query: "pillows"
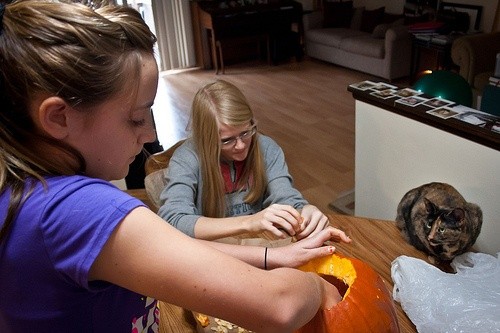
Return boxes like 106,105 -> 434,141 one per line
360,6 -> 385,32
373,18 -> 405,40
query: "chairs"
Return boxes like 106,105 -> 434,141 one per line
145,137 -> 191,221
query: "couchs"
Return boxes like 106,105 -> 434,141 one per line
451,31 -> 500,90
304,10 -> 412,84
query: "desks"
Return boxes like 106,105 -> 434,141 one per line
159,213 -> 453,333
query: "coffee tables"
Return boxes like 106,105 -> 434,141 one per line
417,41 -> 450,78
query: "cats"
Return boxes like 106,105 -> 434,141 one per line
395,182 -> 483,268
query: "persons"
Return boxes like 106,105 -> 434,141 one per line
157,79 -> 329,240
0,0 -> 352,333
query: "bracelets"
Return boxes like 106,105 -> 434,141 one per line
264,247 -> 267,270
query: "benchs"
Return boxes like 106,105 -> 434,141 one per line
210,36 -> 270,74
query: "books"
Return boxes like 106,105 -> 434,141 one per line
408,22 -> 441,36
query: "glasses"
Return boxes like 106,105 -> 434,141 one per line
215,127 -> 254,150
